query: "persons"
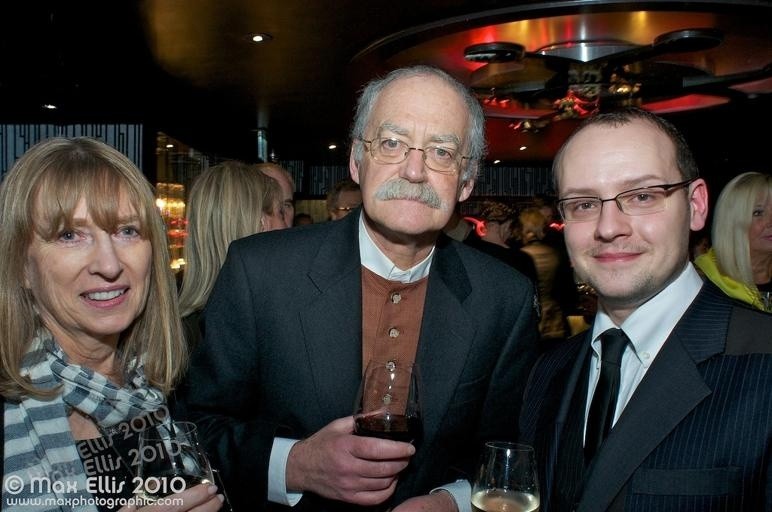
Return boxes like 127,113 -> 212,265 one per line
0,136 -> 225,512
514,105 -> 772,512
172,63 -> 542,512
444,194 -> 572,338
292,213 -> 313,226
326,179 -> 363,222
711,171 -> 772,292
252,163 -> 295,229
178,161 -> 289,318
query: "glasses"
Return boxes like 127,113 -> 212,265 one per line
557,179 -> 694,222
359,135 -> 472,173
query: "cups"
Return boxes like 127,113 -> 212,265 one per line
136,418 -> 219,503
467,438 -> 543,512
353,354 -> 425,451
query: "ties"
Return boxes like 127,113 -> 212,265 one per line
584,329 -> 630,468
